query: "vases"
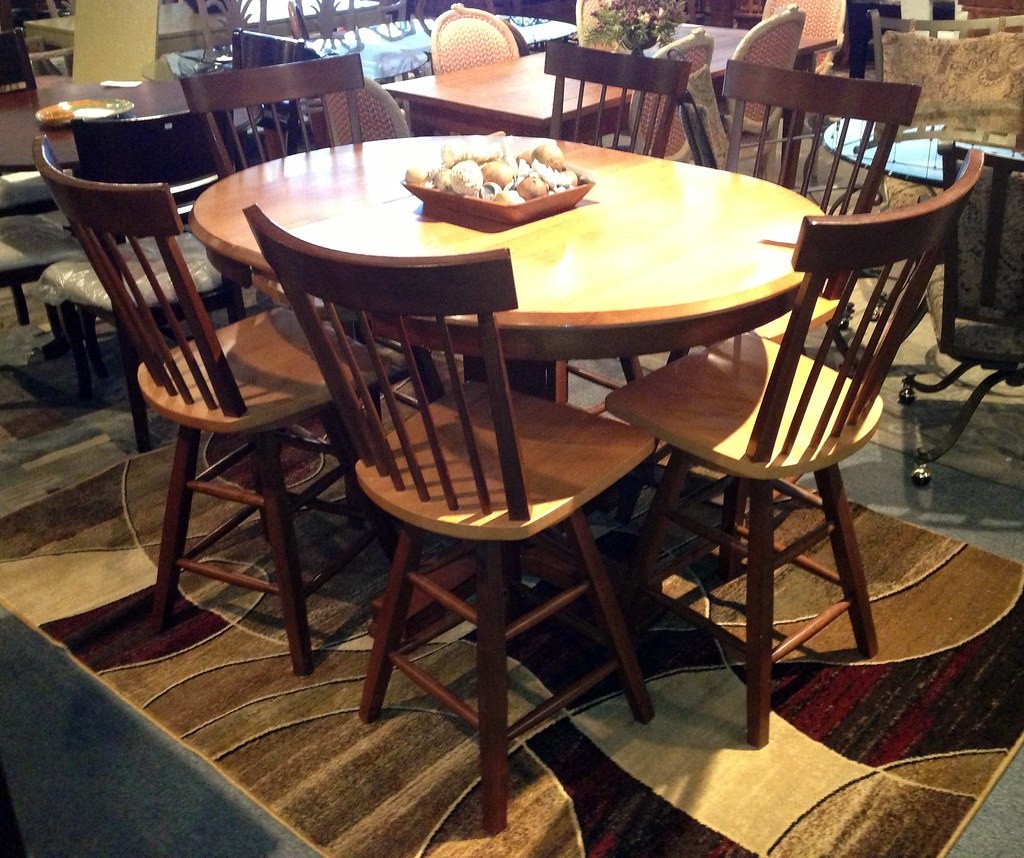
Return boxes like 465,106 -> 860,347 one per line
624,28 -> 660,57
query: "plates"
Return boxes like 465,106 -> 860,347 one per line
34,99 -> 135,127
400,176 -> 595,225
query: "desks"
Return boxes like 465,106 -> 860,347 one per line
174,13 -> 577,149
378,16 -> 839,192
0,81 -> 264,246
23,0 -> 396,96
816,99 -> 1024,363
189,134 -> 827,628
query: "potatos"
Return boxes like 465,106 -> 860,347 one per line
406,144 -> 578,208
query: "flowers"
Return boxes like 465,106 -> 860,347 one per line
579,0 -> 691,56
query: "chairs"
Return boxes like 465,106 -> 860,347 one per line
0,0 -> 1024,847
